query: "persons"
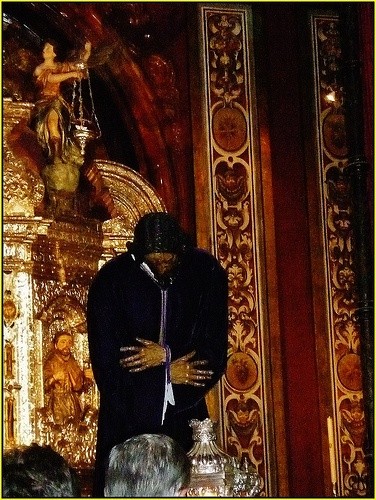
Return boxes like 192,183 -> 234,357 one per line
87,213 -> 229,499
44,331 -> 94,426
103,434 -> 191,498
0,442 -> 80,497
33,40 -> 91,163
